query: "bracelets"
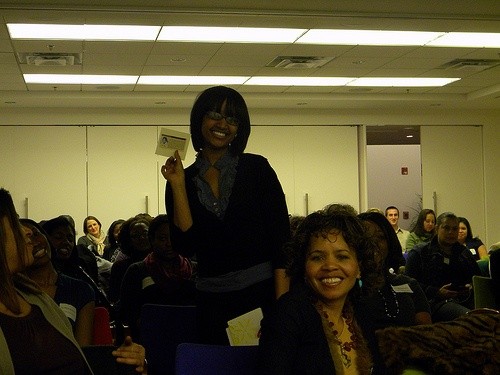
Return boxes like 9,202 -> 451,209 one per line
145,358 -> 149,368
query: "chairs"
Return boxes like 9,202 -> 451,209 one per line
472,259 -> 500,309
92,304 -> 260,375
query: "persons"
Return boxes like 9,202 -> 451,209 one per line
155,86 -> 291,347
257,212 -> 383,374
19,206 -> 488,350
0,188 -> 149,375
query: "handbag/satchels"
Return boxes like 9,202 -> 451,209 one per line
80,345 -> 141,375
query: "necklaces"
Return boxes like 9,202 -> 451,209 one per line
377,287 -> 400,318
322,301 -> 360,351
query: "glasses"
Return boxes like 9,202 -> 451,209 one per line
205,111 -> 240,126
